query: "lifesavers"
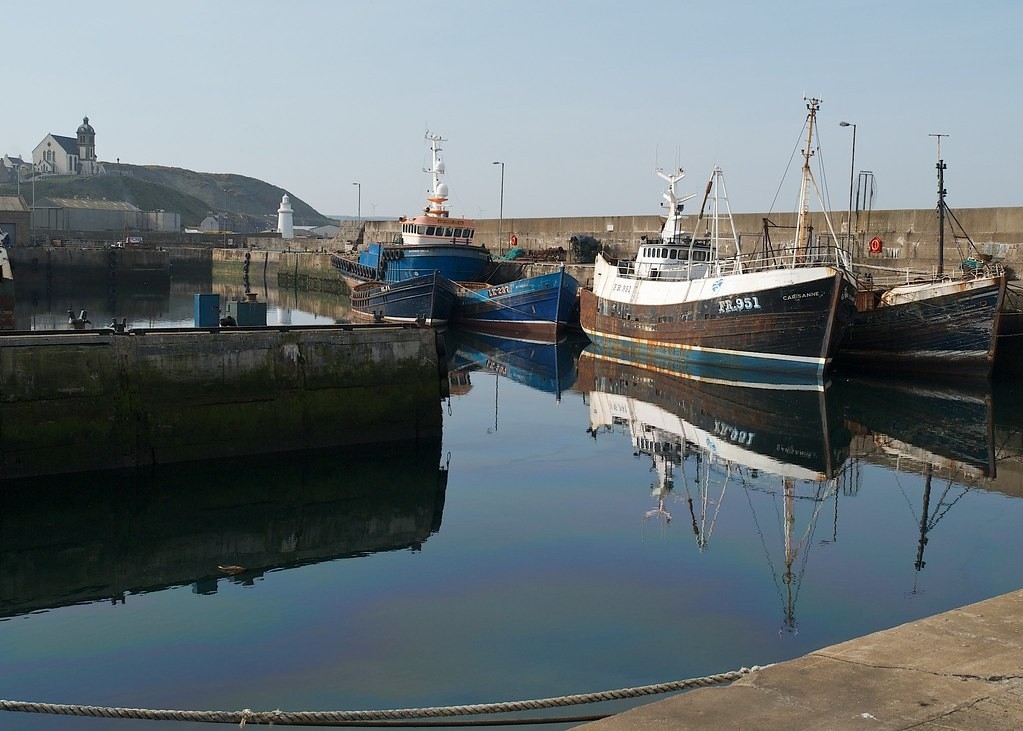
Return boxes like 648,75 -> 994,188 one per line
386,250 -> 389,260
380,260 -> 385,270
107,285 -> 119,304
32,258 -> 38,264
243,273 -> 251,294
378,270 -> 383,279
381,250 -> 386,260
108,250 -> 119,281
330,255 -> 376,280
395,250 -> 401,260
871,239 -> 879,251
512,237 -> 515,244
390,249 -> 394,260
243,252 -> 250,272
837,299 -> 858,324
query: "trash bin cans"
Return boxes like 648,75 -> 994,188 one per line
571,236 -> 595,264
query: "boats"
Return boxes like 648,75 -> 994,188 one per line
573,345 -> 854,640
577,89 -> 859,393
328,128 -> 493,293
445,329 -> 586,406
835,370 -> 998,602
350,269 -> 462,329
842,134 -> 1007,378
449,265 -> 580,344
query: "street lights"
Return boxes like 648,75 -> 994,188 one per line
353,183 -> 361,221
494,162 -> 504,257
224,190 -> 227,248
32,163 -> 37,247
840,121 -> 855,264
